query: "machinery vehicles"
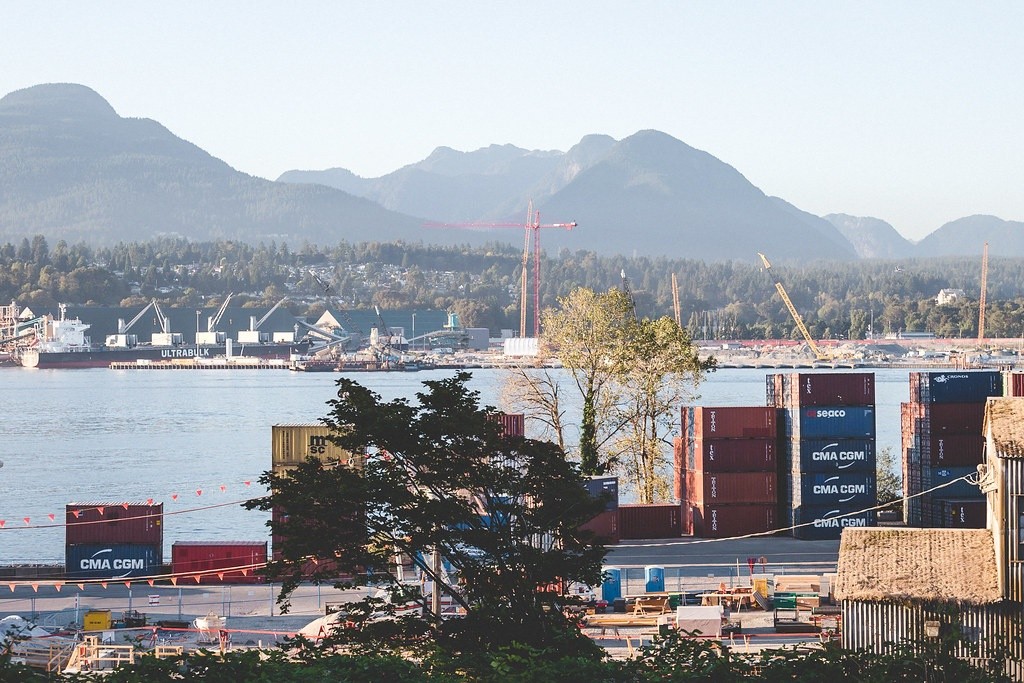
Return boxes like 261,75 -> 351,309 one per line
756,252 -> 836,362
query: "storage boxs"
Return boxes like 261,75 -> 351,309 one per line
273,413 -> 524,581
66,502 -> 163,579
672,407 -> 778,540
564,475 -> 620,546
902,371 -> 1024,528
172,541 -> 268,585
618,503 -> 682,538
766,372 -> 878,540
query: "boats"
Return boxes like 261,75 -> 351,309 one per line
294,360 -> 438,372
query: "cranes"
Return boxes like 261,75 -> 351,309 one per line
420,199 -> 577,338
671,273 -> 682,329
976,241 -> 989,351
